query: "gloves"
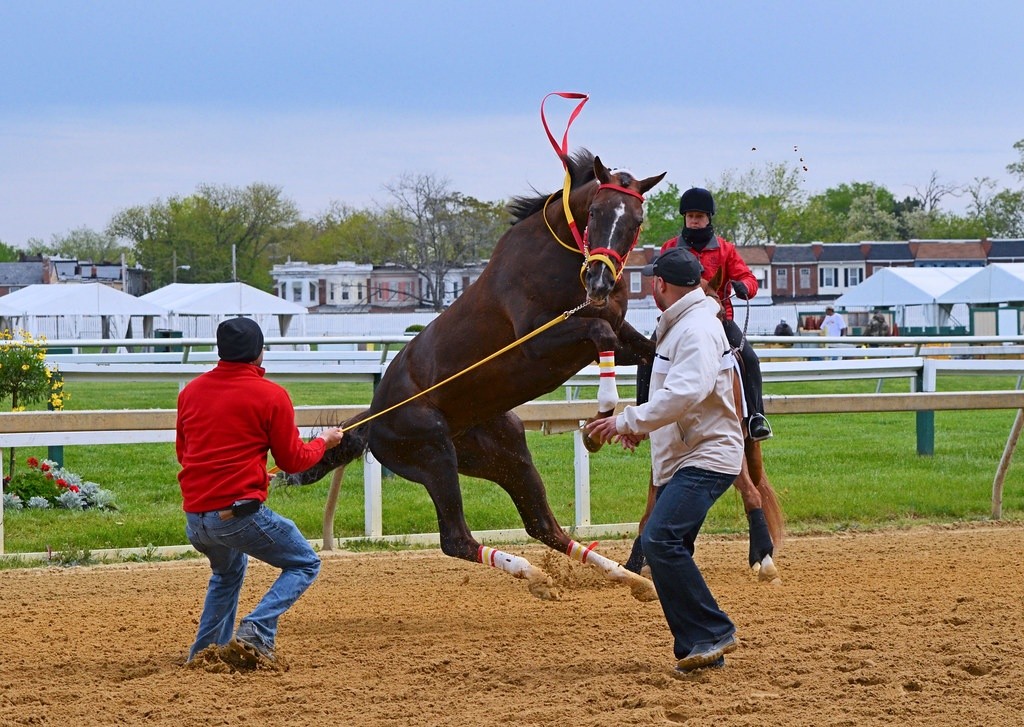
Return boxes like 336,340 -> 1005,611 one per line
731,280 -> 748,299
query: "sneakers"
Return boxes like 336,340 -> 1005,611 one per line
678,635 -> 737,668
238,622 -> 290,671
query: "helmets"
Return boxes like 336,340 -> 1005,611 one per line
678,188 -> 715,215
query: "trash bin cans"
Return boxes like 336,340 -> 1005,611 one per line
774,323 -> 794,335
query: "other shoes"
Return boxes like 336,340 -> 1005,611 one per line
751,419 -> 770,437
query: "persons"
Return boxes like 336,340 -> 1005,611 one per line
635,188 -> 769,439
774,319 -> 794,346
585,247 -> 744,668
176,319 -> 344,667
820,305 -> 846,360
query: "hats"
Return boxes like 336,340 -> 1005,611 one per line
217,317 -> 263,363
642,248 -> 701,285
781,318 -> 786,324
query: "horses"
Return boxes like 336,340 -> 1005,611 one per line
276,147 -> 670,604
636,264 -> 783,585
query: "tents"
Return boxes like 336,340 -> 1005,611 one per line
834,263 -> 1024,337
0,283 -> 307,339
